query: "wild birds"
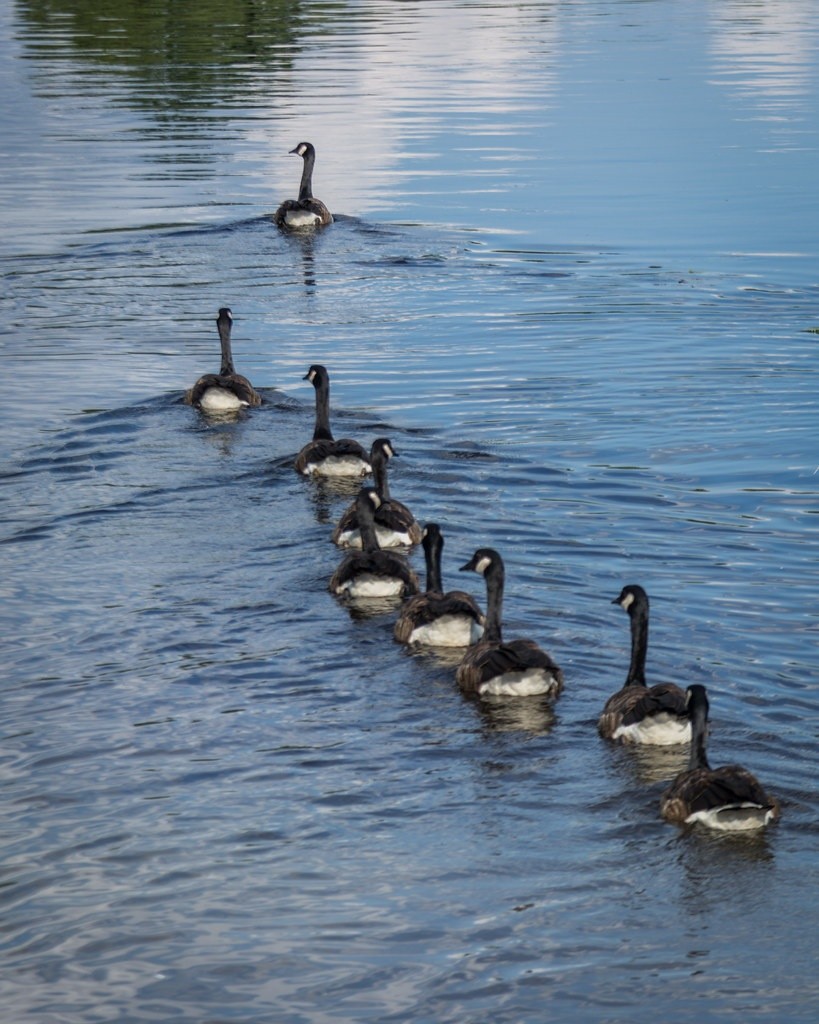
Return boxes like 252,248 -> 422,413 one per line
274,142 -> 334,235
326,437 -> 487,649
294,365 -> 373,479
192,308 -> 263,418
455,547 -> 565,706
596,584 -> 687,748
659,684 -> 776,831
